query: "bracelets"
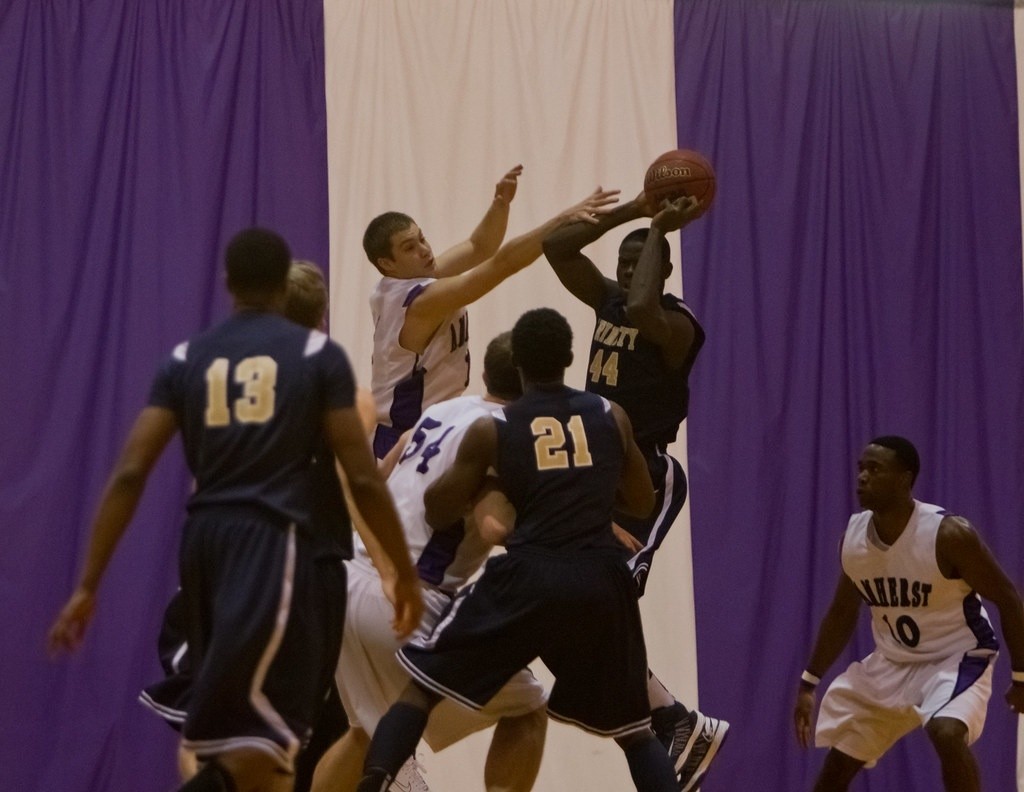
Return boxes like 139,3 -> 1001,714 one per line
1010,670 -> 1024,682
801,670 -> 820,685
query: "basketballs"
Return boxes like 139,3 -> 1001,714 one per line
641,147 -> 721,219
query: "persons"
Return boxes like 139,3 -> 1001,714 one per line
791,434 -> 1024,792
46,164 -> 733,791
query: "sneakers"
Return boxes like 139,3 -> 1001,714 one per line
668,709 -> 730,792
388,754 -> 431,792
